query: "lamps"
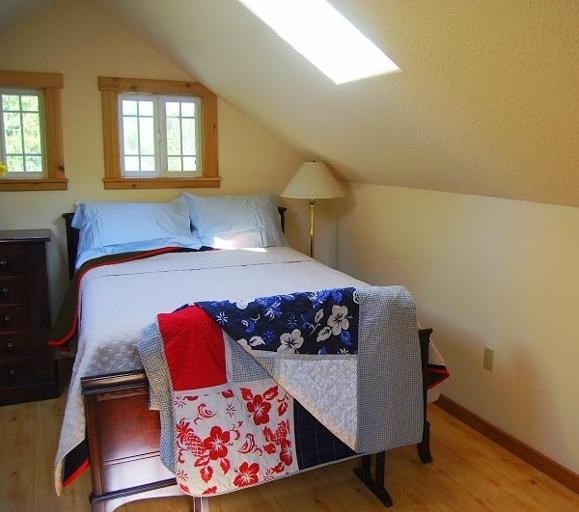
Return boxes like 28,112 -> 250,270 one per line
279,160 -> 347,257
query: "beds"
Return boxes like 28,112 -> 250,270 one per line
61,207 -> 433,512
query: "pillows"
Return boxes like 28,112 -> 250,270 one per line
70,192 -> 288,271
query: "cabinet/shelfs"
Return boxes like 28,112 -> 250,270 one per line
0,239 -> 63,406
